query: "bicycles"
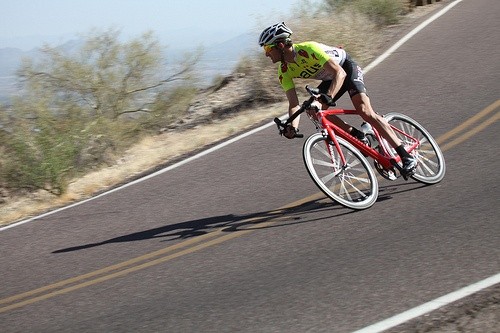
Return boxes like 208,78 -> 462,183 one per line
274,85 -> 447,211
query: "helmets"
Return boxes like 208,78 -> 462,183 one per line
258,21 -> 293,47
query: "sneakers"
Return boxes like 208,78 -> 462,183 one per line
402,154 -> 420,177
360,133 -> 371,157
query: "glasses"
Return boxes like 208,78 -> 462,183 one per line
263,43 -> 278,52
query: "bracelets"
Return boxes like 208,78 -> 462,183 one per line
327,94 -> 334,100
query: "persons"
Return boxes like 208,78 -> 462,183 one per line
258,21 -> 419,176
